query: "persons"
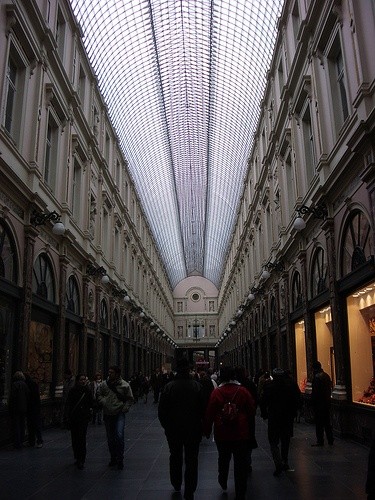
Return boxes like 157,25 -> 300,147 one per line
126,367 -> 179,405
8,371 -> 29,451
193,364 -> 300,448
91,371 -> 104,426
24,372 -> 43,449
158,360 -> 207,500
309,362 -> 336,445
261,368 -> 301,476
205,367 -> 256,499
98,366 -> 133,470
62,369 -> 76,430
66,374 -> 94,470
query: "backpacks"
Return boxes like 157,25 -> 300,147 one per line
215,386 -> 241,425
